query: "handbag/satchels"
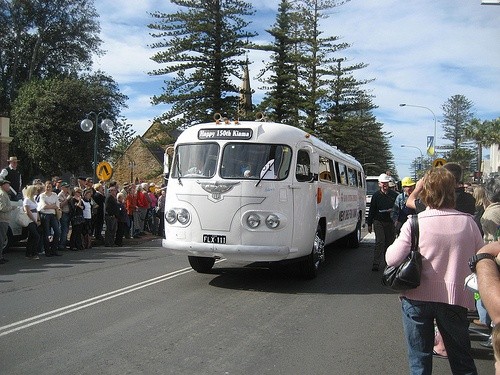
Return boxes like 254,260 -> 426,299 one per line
381,215 -> 421,292
16,211 -> 29,228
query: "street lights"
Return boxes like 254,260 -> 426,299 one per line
399,103 -> 439,170
400,145 -> 424,181
80,111 -> 116,236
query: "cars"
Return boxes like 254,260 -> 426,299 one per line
0,185 -> 30,250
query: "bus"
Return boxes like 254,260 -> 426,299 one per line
357,176 -> 396,224
160,112 -> 367,274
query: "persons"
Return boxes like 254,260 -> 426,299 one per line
366,173 -> 400,272
0,154 -> 168,270
385,167 -> 500,375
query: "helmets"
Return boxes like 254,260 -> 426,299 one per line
402,177 -> 416,187
377,173 -> 390,182
